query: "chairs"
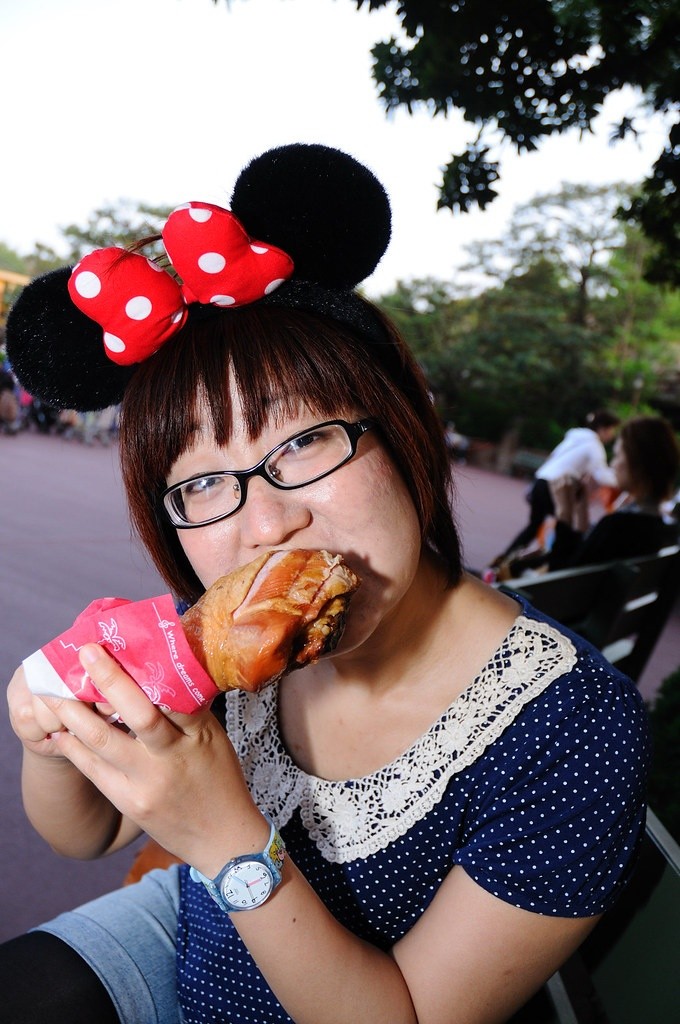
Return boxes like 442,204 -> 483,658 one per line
505,803 -> 680,1024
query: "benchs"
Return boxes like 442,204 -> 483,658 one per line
479,544 -> 680,688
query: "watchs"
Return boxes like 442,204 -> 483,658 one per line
187,817 -> 286,911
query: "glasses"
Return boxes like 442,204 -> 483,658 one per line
157,418 -> 378,530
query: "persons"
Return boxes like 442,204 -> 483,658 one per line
487,405 -> 680,580
444,417 -> 470,456
0,333 -> 121,445
0,144 -> 646,1024
